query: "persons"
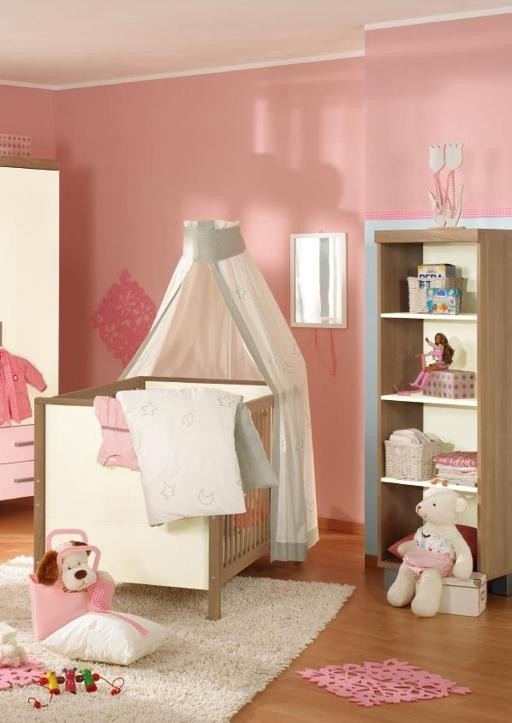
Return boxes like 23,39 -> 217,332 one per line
409,333 -> 455,390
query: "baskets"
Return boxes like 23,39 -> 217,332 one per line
384,435 -> 442,480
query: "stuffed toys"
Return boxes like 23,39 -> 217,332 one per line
38,541 -> 112,593
387,487 -> 473,618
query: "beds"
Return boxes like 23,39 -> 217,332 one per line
32,375 -> 276,620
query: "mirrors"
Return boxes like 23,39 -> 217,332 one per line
289,232 -> 348,330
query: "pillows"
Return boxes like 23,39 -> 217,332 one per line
37,608 -> 174,666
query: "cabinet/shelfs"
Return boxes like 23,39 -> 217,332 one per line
372,227 -> 510,594
0,162 -> 60,503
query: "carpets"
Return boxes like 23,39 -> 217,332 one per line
0,554 -> 358,723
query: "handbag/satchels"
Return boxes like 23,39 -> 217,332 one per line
29,527 -> 115,639
